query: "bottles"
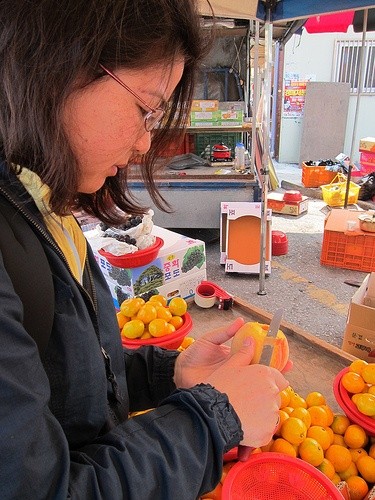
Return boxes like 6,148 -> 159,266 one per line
234,143 -> 245,171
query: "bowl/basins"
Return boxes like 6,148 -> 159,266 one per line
98,237 -> 164,268
333,365 -> 375,438
357,213 -> 375,232
283,190 -> 302,201
272,230 -> 288,255
119,305 -> 192,351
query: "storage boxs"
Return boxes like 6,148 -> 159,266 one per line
302,137 -> 375,188
220,202 -> 272,274
320,209 -> 375,273
154,99 -> 245,161
341,272 -> 375,364
267,193 -> 310,215
81,225 -> 206,314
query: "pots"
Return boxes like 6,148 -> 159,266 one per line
212,142 -> 232,158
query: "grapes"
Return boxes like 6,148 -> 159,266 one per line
99,215 -> 143,245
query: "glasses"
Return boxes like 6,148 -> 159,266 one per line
99,62 -> 164,134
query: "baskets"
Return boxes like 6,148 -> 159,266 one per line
272,230 -> 289,256
333,362 -> 375,438
98,236 -> 164,269
319,173 -> 361,207
284,190 -> 302,202
358,211 -> 375,233
114,305 -> 193,351
222,452 -> 345,500
223,416 -> 281,463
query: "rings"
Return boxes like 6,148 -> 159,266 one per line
276,416 -> 281,426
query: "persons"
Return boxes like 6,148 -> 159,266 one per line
0,0 -> 293,500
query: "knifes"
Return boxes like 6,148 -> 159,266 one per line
237,308 -> 284,463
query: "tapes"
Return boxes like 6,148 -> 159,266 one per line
194,283 -> 217,309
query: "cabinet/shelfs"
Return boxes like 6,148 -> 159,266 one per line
116,68 -> 262,228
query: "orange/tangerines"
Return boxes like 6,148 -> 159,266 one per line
200,385 -> 375,500
342,359 -> 375,418
116,295 -> 195,352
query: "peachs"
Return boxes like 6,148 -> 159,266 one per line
230,322 -> 289,373
